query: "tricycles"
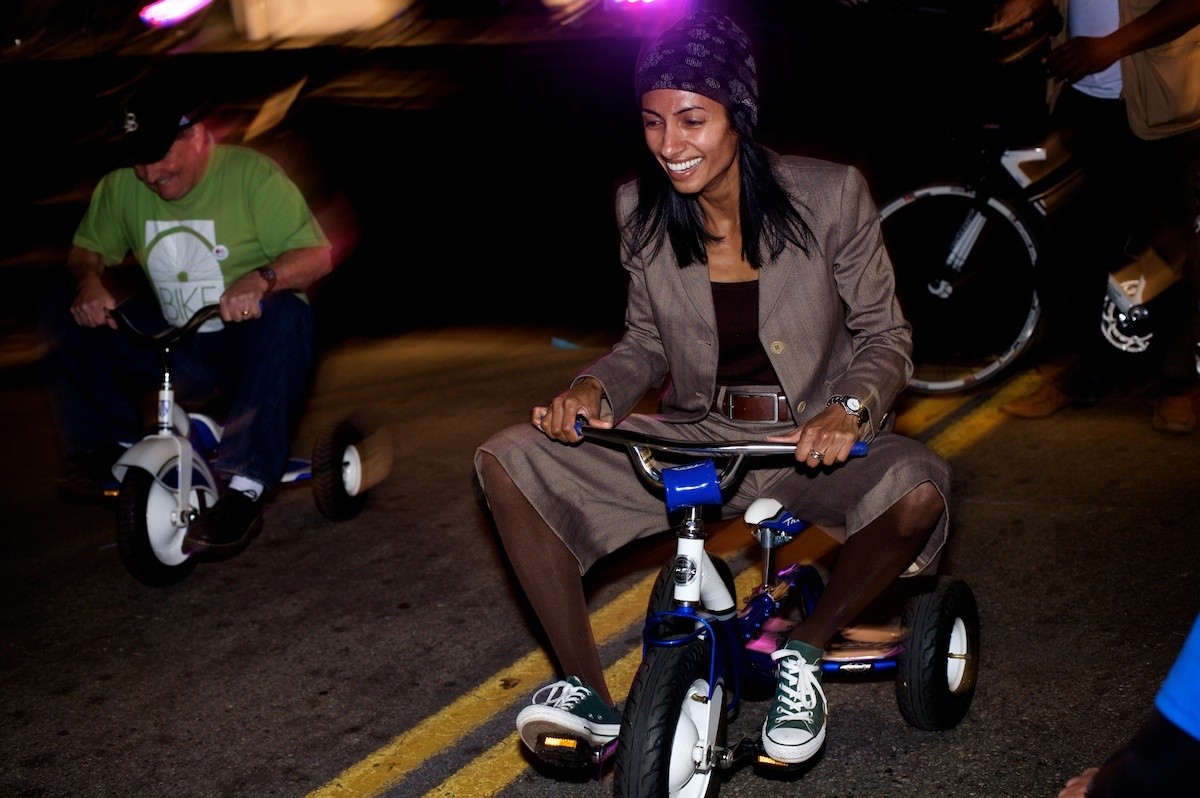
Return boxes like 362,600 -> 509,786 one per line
101,298 -> 371,585
531,410 -> 984,798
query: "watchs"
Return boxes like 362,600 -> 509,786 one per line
831,394 -> 866,418
255,264 -> 277,293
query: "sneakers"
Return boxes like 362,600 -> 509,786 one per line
514,675 -> 624,758
760,639 -> 830,769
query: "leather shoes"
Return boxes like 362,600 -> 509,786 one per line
178,486 -> 276,557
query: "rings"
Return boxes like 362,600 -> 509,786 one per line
241,309 -> 251,315
809,448 -> 825,463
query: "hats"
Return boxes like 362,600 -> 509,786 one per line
104,77 -> 180,165
634,7 -> 761,126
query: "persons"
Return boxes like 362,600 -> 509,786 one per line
65,78 -> 335,560
993,1 -> 1200,431
1057,607 -> 1200,798
472,7 -> 956,765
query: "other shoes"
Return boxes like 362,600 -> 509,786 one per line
1154,380 -> 1199,435
999,380 -> 1079,418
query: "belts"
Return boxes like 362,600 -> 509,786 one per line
706,387 -> 804,432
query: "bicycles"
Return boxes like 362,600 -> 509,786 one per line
860,46 -> 1200,397
